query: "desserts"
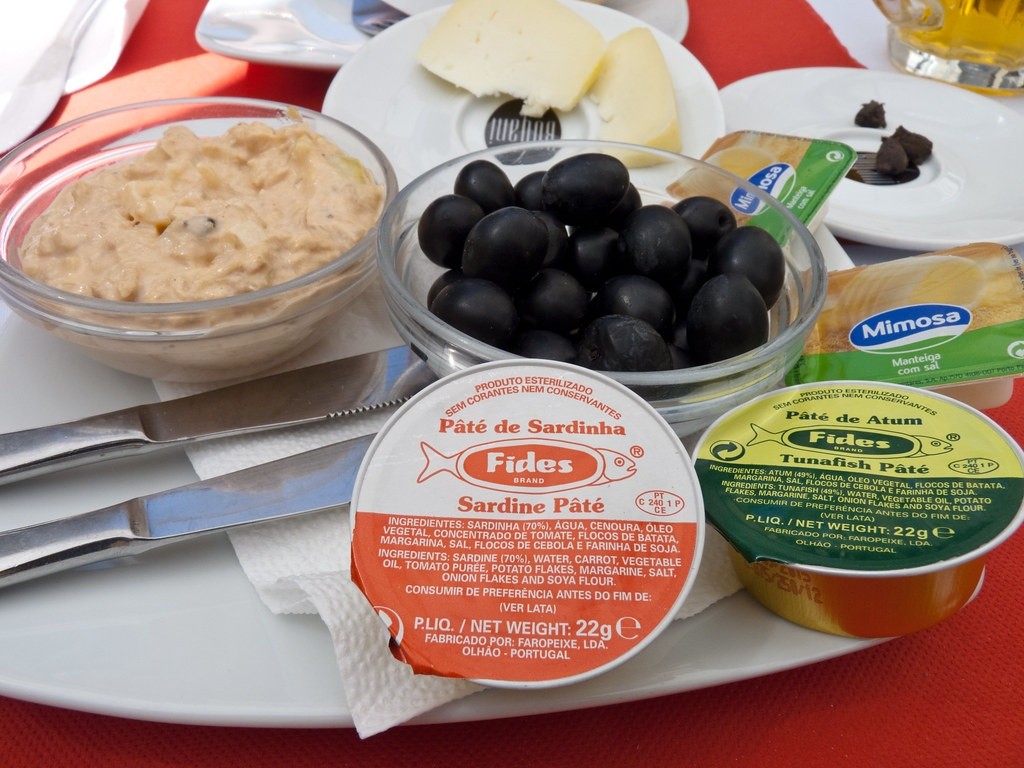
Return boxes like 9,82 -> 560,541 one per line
20,113 -> 386,374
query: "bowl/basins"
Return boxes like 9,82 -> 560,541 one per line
0,94 -> 396,382
374,140 -> 828,438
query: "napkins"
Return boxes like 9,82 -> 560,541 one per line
0,0 -> 149,108
178,389 -> 744,741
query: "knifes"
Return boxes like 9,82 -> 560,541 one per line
0,0 -> 105,153
1,347 -> 432,485
1,430 -> 376,582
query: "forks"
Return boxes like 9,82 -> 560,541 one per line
353,1 -> 410,33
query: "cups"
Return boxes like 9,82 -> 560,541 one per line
876,0 -> 1024,96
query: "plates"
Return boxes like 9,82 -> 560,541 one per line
719,66 -> 1024,249
318,4 -> 725,233
194,2 -> 689,68
1,292 -> 987,729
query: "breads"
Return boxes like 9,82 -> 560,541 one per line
418,1 -> 603,116
583,27 -> 682,166
